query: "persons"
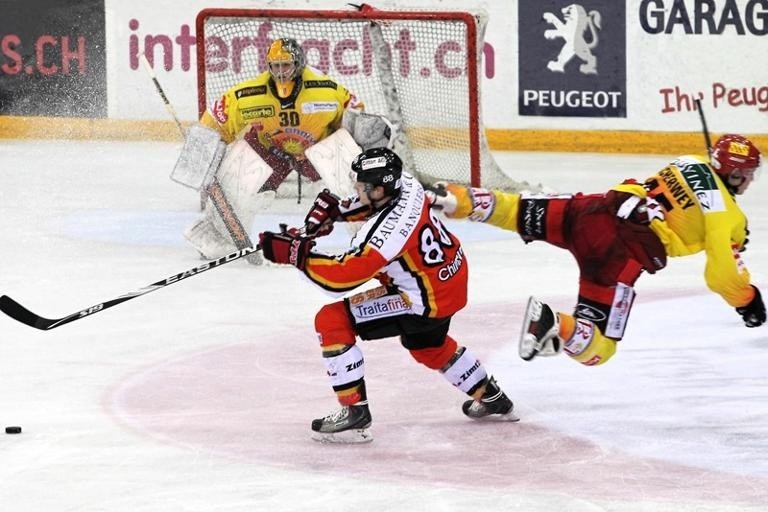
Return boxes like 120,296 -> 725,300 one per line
168,39 -> 413,261
256,147 -> 519,444
420,135 -> 768,368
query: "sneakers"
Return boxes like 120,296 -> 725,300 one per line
461,376 -> 514,420
253,183 -> 277,210
311,402 -> 373,434
518,294 -> 565,362
424,180 -> 458,215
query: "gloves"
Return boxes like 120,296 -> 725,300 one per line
304,190 -> 340,240
735,283 -> 767,328
256,223 -> 317,274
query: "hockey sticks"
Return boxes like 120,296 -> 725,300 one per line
690,78 -> 759,322
1,224 -> 310,329
148,76 -> 273,266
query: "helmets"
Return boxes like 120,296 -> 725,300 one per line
709,133 -> 762,177
349,146 -> 404,198
266,37 -> 306,84
